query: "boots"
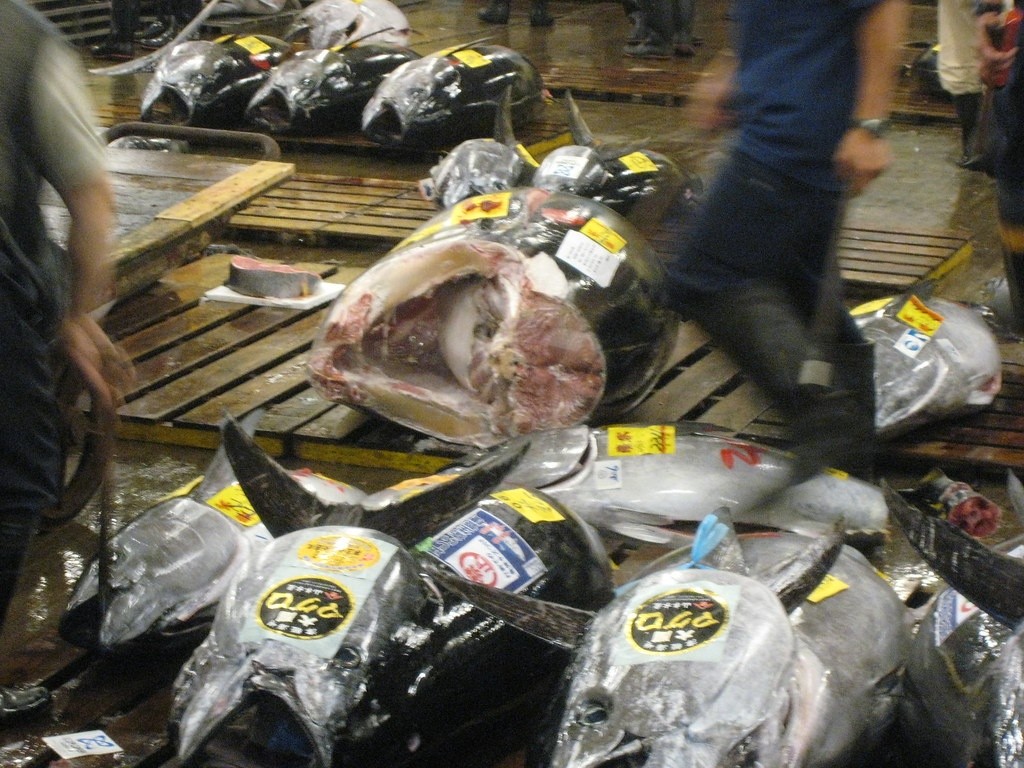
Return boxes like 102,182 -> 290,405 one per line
785,342 -> 890,571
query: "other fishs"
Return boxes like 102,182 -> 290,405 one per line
847,278 -> 1003,438
302,82 -> 700,449
58,404 -> 1024,768
137,0 -> 543,150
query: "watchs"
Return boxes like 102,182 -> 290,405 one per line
848,117 -> 890,136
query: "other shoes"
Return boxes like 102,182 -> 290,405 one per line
622,35 -> 672,59
675,30 -> 705,55
529,7 -> 554,26
476,3 -> 510,23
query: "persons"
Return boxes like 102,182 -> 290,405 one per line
936,0 -> 1024,330
660,0 -> 909,480
88,0 -> 202,61
0,0 -> 127,619
478,0 -> 556,26
622,0 -> 704,59
